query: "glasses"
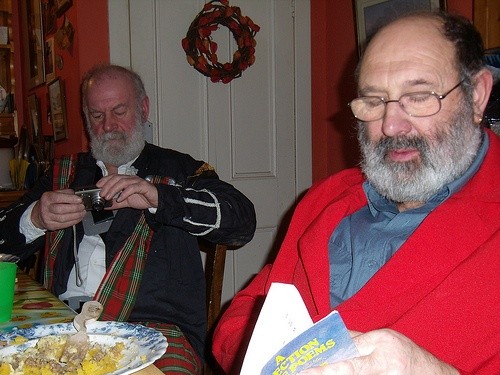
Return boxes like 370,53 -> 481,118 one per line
348,79 -> 468,122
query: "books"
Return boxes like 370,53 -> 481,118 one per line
240,282 -> 360,374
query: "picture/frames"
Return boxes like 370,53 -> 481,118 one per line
25,0 -> 68,144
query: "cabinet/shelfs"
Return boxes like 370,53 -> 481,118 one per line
0,0 -> 19,149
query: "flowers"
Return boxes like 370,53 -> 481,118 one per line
182,0 -> 261,84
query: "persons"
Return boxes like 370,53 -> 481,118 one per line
210,11 -> 499,375
0,64 -> 257,363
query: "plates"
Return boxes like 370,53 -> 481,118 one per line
0,321 -> 169,375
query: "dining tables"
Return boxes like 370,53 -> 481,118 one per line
0,271 -> 167,375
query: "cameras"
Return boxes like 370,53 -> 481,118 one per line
74,185 -> 113,213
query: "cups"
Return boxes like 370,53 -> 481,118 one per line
0,262 -> 20,326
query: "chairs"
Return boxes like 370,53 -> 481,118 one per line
198,244 -> 227,375
